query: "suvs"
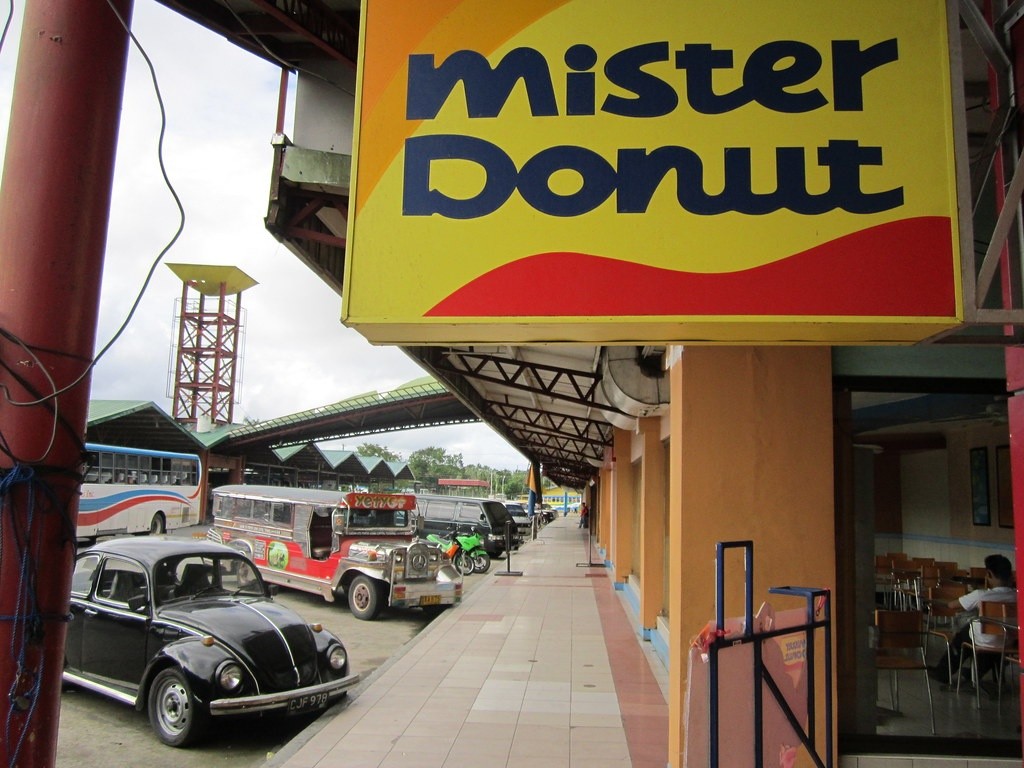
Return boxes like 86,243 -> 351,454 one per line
60,536 -> 362,748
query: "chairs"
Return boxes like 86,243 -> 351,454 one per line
84,470 -> 187,486
312,527 -> 332,558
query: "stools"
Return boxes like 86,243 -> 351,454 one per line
873,552 -> 1018,734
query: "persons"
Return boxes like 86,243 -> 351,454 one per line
927,554 -> 1017,684
577,502 -> 588,530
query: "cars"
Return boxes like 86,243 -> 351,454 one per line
413,493 -> 559,559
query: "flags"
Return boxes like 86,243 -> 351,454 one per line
526,462 -> 538,519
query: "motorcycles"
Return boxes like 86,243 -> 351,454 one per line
426,522 -> 490,576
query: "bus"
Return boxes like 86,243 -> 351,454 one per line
76,443 -> 203,544
207,484 -> 465,621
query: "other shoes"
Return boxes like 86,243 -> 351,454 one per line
925,666 -> 949,686
961,667 -> 979,683
